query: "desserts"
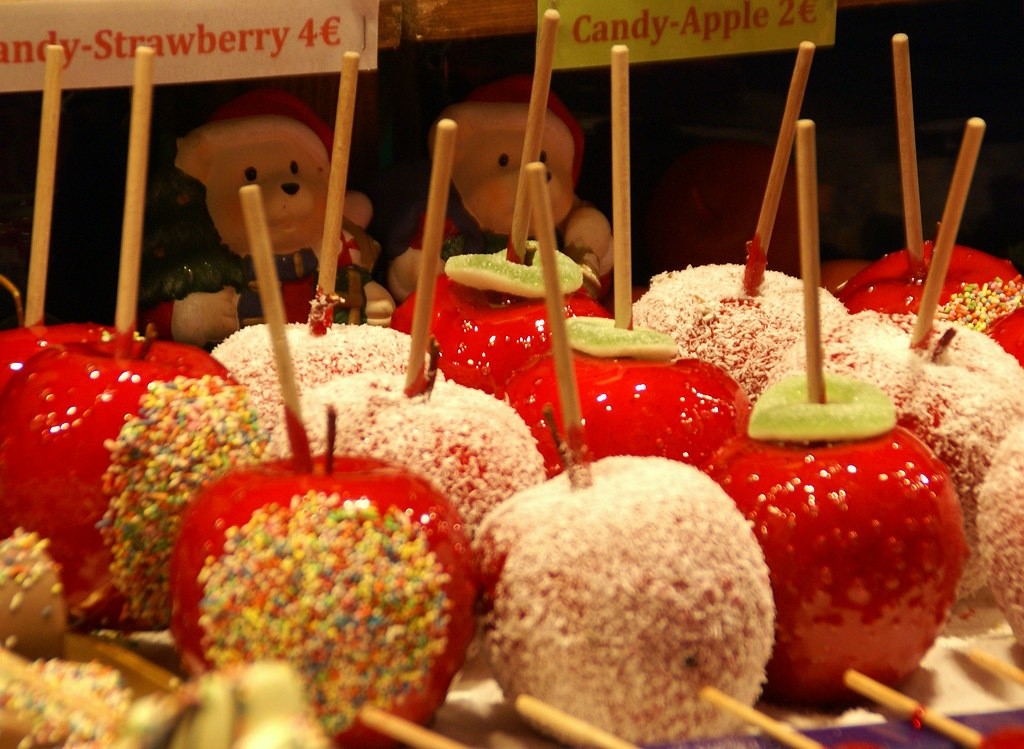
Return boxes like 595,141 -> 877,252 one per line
0,6 -> 1024,749
